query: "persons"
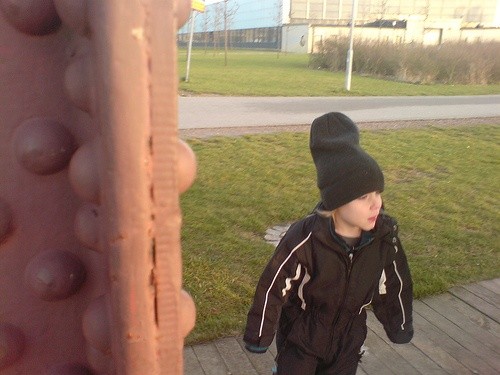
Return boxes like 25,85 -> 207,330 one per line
242,111 -> 415,375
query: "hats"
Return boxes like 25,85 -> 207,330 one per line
309,111 -> 384,211
